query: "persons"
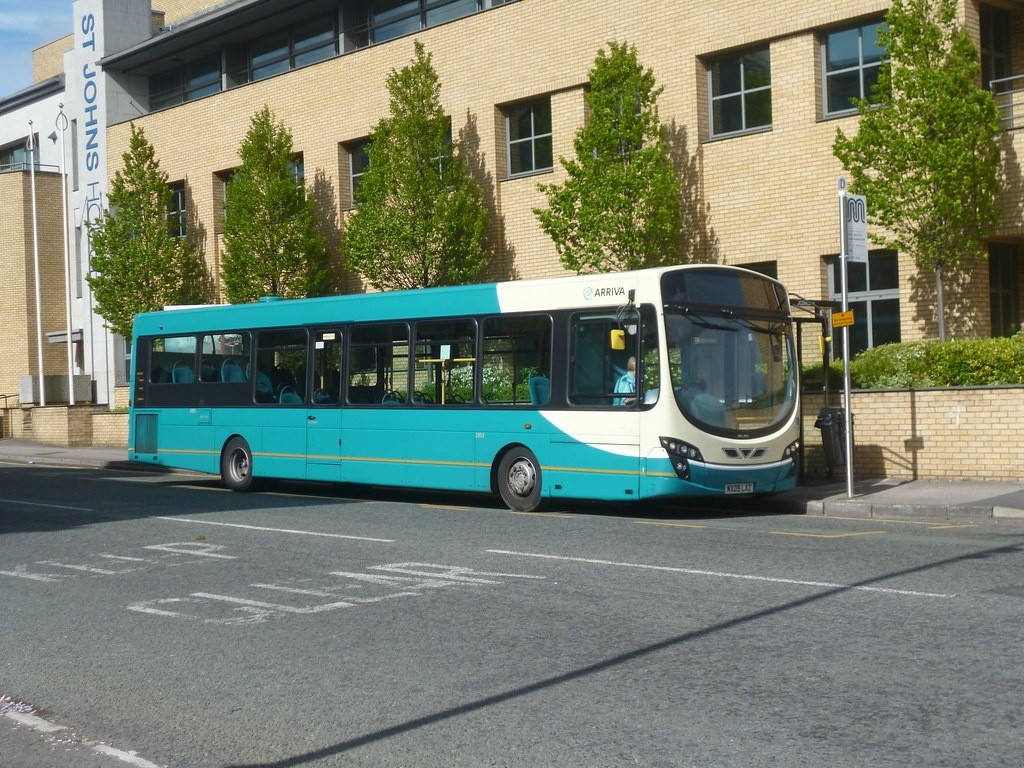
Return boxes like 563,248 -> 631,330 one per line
612,355 -> 637,406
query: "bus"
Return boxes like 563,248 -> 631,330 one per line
127,263 -> 830,513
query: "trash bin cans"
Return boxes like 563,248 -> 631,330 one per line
818,405 -> 856,481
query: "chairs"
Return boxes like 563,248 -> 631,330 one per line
172,360 -> 193,384
220,358 -> 245,382
246,361 -> 331,405
376,389 -> 465,405
528,376 -> 550,405
201,359 -> 217,381
